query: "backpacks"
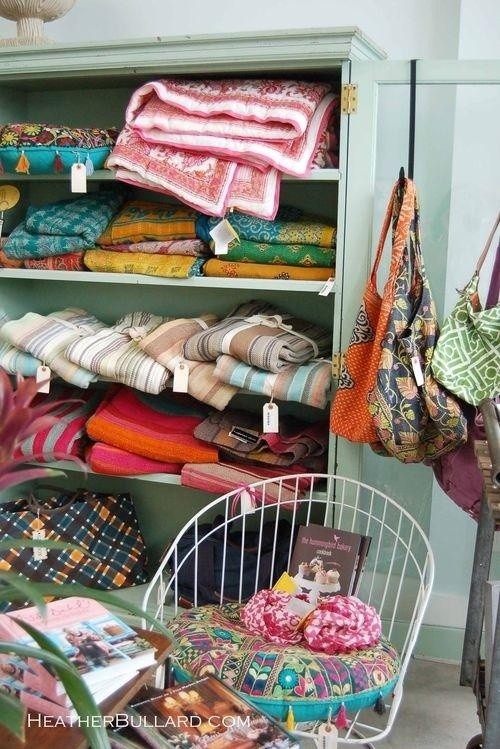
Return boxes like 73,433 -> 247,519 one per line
160,514 -> 299,608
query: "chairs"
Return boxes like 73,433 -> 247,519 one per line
133,470 -> 437,749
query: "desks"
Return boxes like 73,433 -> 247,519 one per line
0,595 -> 179,749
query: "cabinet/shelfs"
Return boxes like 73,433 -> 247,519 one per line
1,23 -> 392,615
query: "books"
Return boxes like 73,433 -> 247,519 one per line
289,525 -> 362,599
350,534 -> 372,597
1,595 -> 299,748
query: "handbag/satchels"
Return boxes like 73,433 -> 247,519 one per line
329,178 -> 499,528
0,484 -> 151,614
